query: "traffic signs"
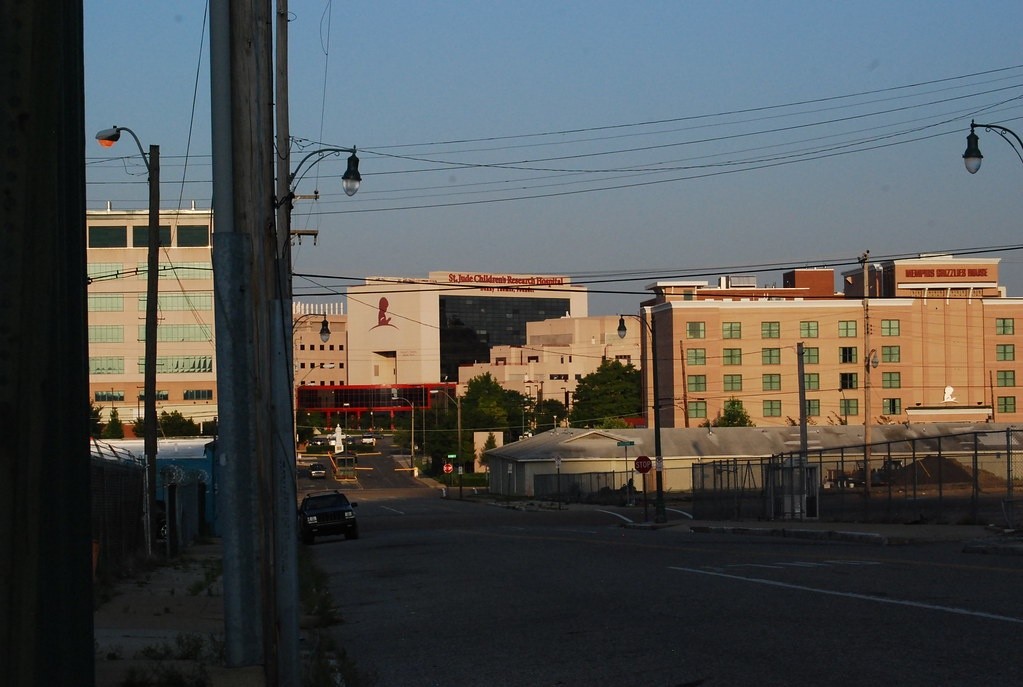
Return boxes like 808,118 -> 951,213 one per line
617,442 -> 634,446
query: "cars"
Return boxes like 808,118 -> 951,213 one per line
346,437 -> 356,445
370,431 -> 384,439
312,438 -> 324,446
298,492 -> 360,541
307,463 -> 328,479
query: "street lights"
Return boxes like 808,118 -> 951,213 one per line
553,415 -> 556,429
838,388 -> 847,425
617,312 -> 666,523
652,404 -> 689,428
562,387 -> 568,428
526,386 -> 531,403
274,146 -> 365,371
863,349 -> 879,525
391,396 -> 414,476
430,390 -> 464,502
344,402 -> 349,472
417,384 -> 425,456
534,384 -> 539,407
97,127 -> 159,577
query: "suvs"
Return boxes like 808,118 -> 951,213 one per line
362,434 -> 374,445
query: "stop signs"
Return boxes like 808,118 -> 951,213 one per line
634,455 -> 652,474
443,464 -> 453,473
